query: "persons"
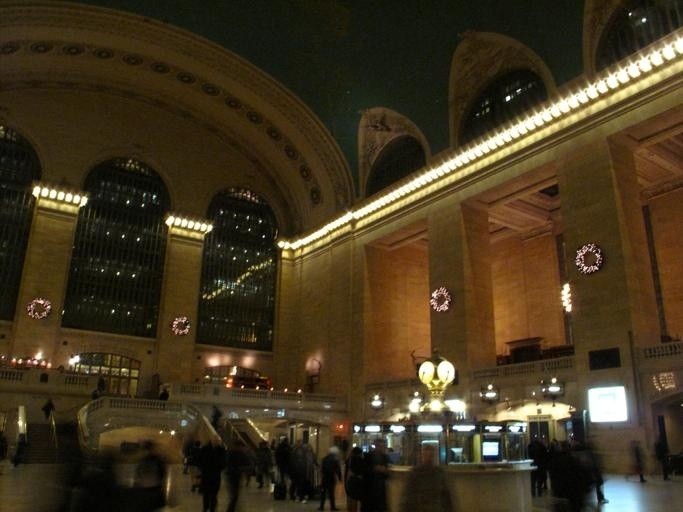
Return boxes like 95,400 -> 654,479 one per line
41,399 -> 55,424
97,375 -> 105,397
212,406 -> 221,430
529,433 -> 604,505
160,389 -> 169,410
0,431 -> 8,475
400,445 -> 453,512
499,425 -> 508,432
518,426 -> 523,432
654,433 -> 672,479
626,441 -> 647,482
91,390 -> 98,407
507,426 -> 511,432
11,434 -> 25,469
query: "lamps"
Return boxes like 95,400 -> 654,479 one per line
418,355 -> 456,412
